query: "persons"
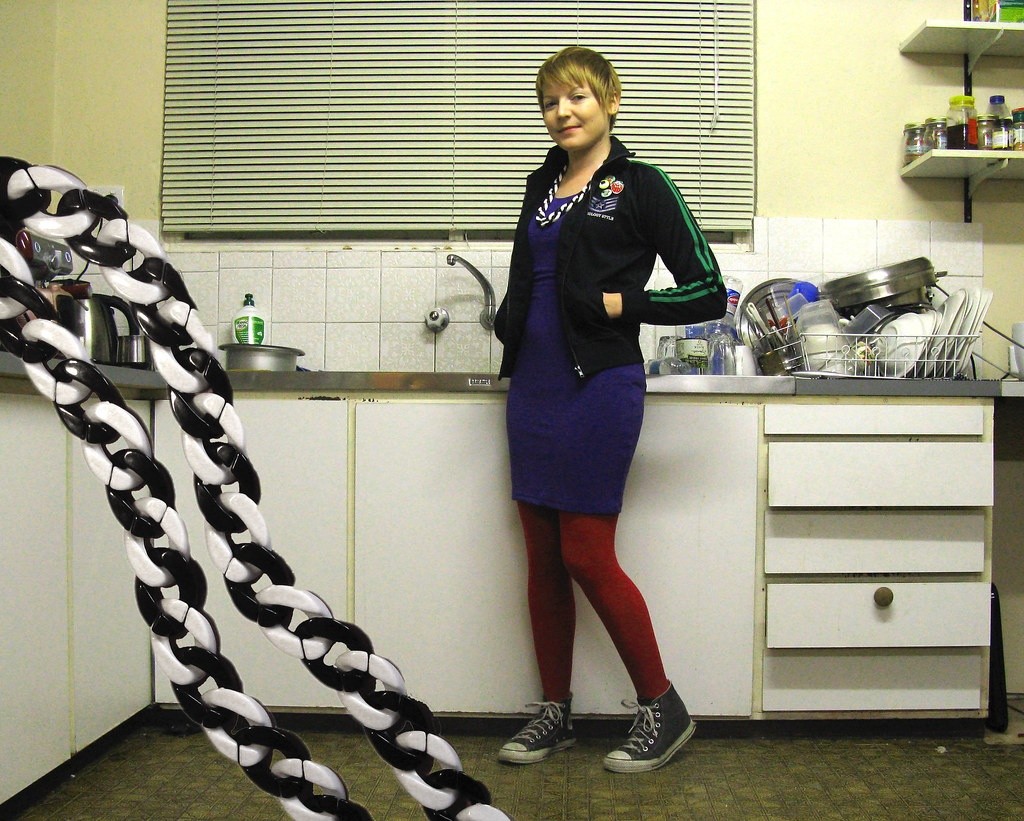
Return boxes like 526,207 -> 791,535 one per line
492,46 -> 728,773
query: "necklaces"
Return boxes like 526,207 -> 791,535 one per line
535,163 -> 591,227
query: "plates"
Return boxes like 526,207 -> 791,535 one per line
875,287 -> 995,377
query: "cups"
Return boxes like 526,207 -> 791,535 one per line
657,335 -> 683,358
116,335 -> 147,366
707,333 -> 737,375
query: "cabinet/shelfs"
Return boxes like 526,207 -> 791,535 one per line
151,398 -> 349,706
354,398 -> 760,722
761,407 -> 995,711
0,392 -> 154,805
896,0 -> 1023,222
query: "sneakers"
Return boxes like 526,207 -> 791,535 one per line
499,693 -> 577,765
603,680 -> 696,775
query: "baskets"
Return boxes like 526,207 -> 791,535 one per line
748,324 -> 981,378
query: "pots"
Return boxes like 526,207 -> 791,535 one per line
816,254 -> 948,308
217,343 -> 306,372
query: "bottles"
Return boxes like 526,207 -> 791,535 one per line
946,93 -> 978,150
716,276 -> 744,332
641,356 -> 692,375
986,95 -> 1011,119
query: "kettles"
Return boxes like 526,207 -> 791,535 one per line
70,293 -> 140,363
